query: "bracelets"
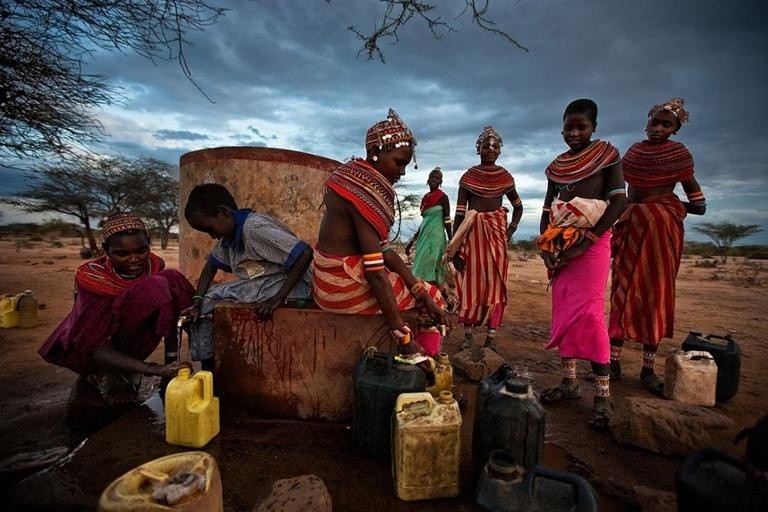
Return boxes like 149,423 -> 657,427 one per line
411,280 -> 429,301
585,228 -> 600,243
190,295 -> 205,303
142,361 -> 159,377
389,323 -> 417,348
411,234 -> 418,243
509,222 -> 519,230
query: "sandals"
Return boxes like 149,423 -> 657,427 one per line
486,338 -> 498,352
541,381 -> 582,400
639,373 -> 663,393
588,396 -> 615,427
586,362 -> 621,382
460,333 -> 473,350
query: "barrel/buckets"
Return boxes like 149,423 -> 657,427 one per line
681,331 -> 741,403
664,349 -> 718,407
421,353 -> 454,398
474,449 -> 600,512
353,349 -> 427,452
473,362 -> 545,477
675,443 -> 768,512
743,412 -> 768,471
165,368 -> 220,449
392,391 -> 463,501
18,290 -> 39,328
0,293 -> 18,328
97,451 -> 224,512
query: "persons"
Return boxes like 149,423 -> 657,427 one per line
305,107 -> 467,409
33,210 -> 197,412
178,182 -> 316,371
538,97 -> 628,430
582,96 -> 708,396
451,125 -> 525,354
404,166 -> 456,317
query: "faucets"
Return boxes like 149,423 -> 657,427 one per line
177,311 -> 212,327
